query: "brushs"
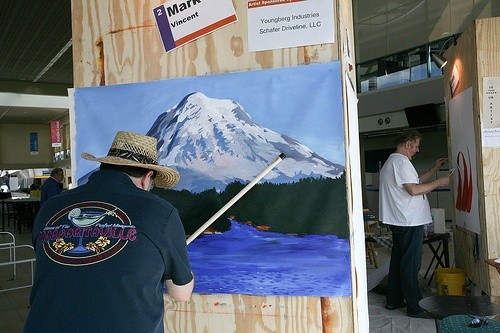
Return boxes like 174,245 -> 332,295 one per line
448,168 -> 454,177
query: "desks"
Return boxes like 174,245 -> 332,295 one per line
0,194 -> 41,235
435,296 -> 500,320
365,232 -> 451,288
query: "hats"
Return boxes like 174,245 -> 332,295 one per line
80,130 -> 181,188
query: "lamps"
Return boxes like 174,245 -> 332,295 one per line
431,33 -> 462,69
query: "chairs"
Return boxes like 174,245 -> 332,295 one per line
6,201 -> 41,234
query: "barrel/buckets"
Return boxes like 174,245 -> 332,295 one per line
434,269 -> 464,296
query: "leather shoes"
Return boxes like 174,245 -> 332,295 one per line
385,301 -> 407,310
407,308 -> 436,319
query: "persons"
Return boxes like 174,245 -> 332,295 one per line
20,131 -> 194,333
378,127 -> 452,320
39,167 -> 64,208
